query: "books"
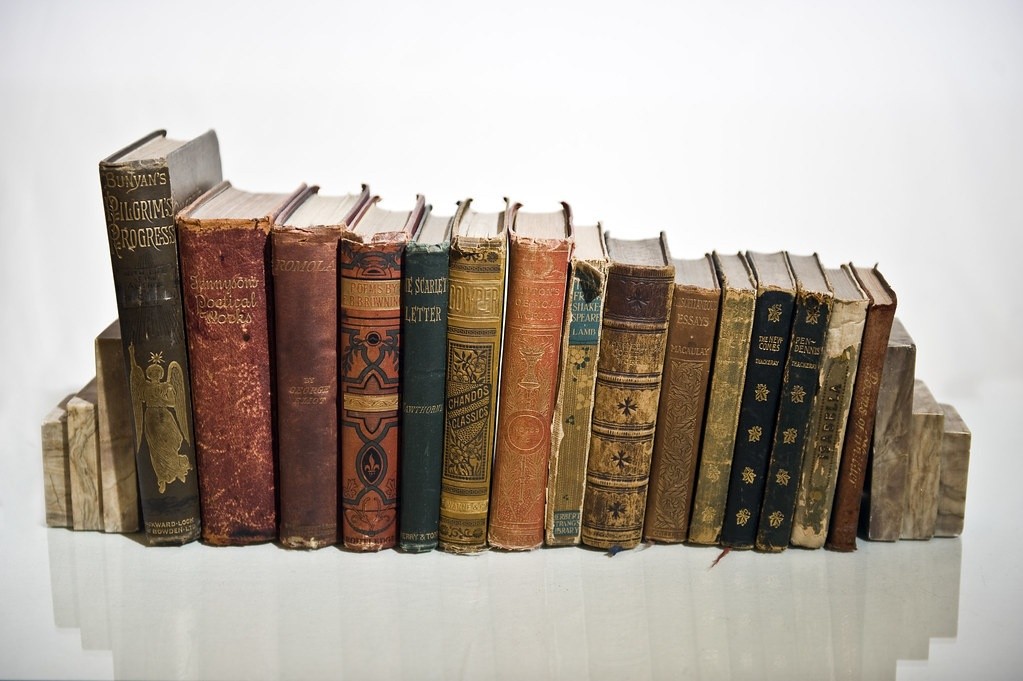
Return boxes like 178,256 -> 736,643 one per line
99,129 -> 898,556
